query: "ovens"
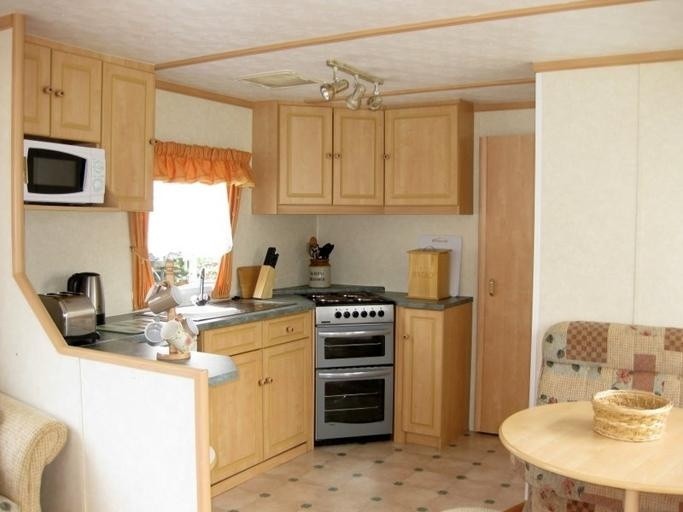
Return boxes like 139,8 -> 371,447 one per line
315,323 -> 394,444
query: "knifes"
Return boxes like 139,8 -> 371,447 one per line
264,248 -> 279,267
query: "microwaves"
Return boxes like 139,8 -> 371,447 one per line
23,140 -> 106,205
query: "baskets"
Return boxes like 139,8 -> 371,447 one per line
592,389 -> 673,442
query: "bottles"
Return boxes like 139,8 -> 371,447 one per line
307,259 -> 332,290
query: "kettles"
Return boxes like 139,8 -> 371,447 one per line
68,272 -> 105,326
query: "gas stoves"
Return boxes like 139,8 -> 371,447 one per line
305,289 -> 394,325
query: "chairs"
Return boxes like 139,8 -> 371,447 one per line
525,318 -> 683,512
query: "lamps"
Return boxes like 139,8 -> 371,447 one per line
315,61 -> 383,110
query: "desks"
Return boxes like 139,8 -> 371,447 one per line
498,396 -> 683,512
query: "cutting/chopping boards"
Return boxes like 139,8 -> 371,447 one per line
417,234 -> 462,297
239,266 -> 261,300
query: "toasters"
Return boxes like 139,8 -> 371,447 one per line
39,290 -> 101,344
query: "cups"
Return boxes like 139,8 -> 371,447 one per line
148,280 -> 182,314
175,317 -> 199,337
145,321 -> 164,346
161,321 -> 196,355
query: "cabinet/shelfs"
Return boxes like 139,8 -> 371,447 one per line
393,302 -> 473,452
199,310 -> 316,498
250,103 -> 385,216
384,100 -> 474,215
19,33 -> 103,145
103,55 -> 156,215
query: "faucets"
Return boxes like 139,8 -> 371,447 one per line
199,268 -> 205,299
